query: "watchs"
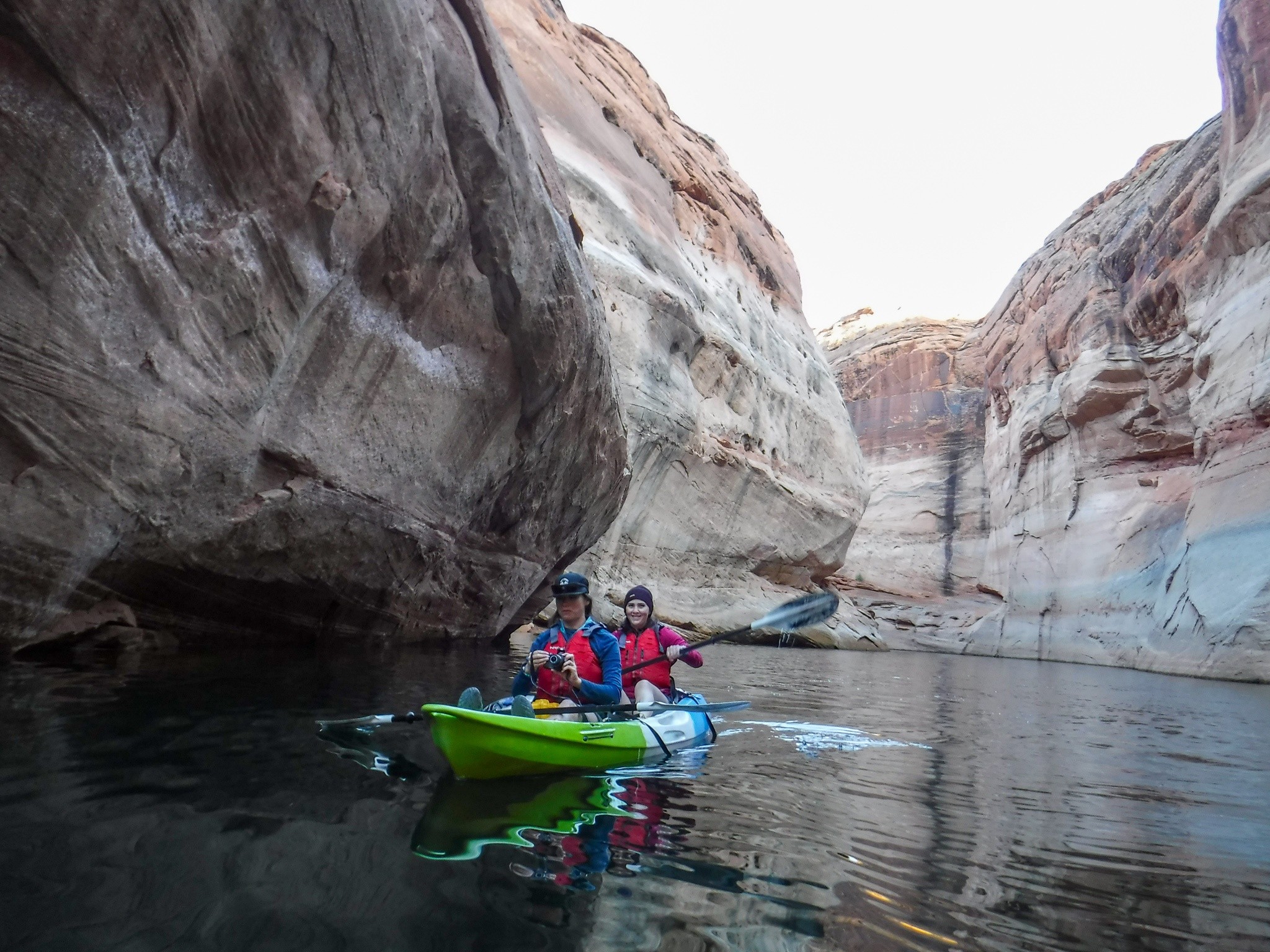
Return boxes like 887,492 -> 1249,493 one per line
523,666 -> 530,677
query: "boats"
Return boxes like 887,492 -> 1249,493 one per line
420,691 -> 719,784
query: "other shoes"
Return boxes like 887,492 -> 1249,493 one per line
458,687 -> 483,712
511,695 -> 536,719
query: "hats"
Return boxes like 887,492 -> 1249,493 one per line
623,585 -> 654,615
547,571 -> 589,596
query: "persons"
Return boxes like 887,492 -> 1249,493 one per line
457,571 -> 622,723
611,585 -> 703,719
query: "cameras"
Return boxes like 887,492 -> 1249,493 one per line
543,648 -> 571,672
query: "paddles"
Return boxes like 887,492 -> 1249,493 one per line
483,592 -> 839,711
315,701 -> 753,729
